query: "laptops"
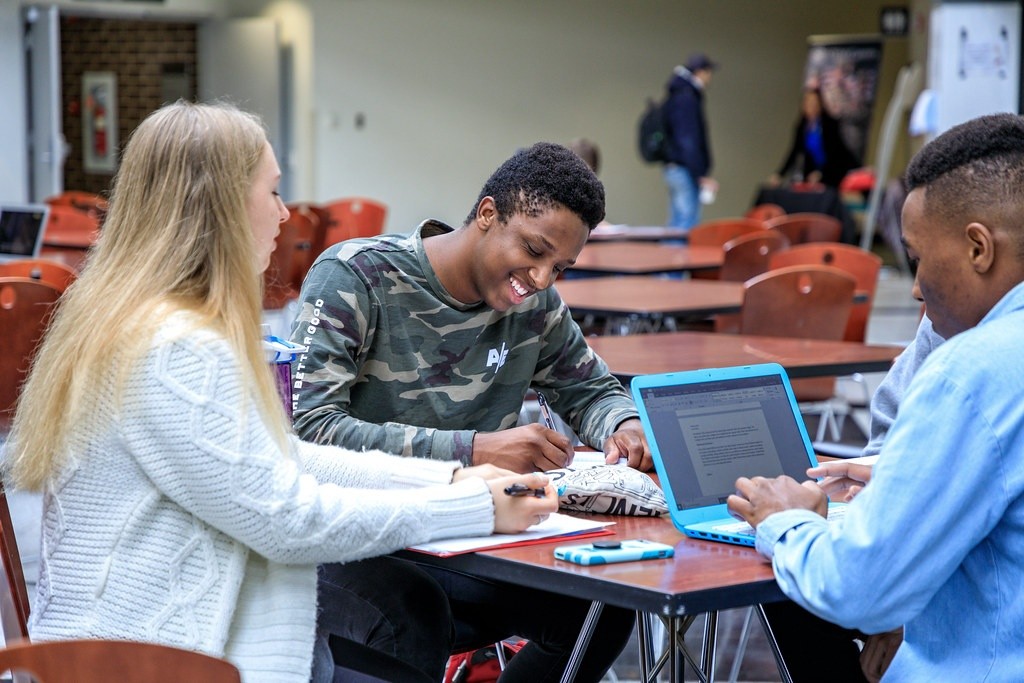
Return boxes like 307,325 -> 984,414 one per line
0,204 -> 50,263
629,362 -> 851,549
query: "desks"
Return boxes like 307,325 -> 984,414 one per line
553,275 -> 746,336
583,331 -> 906,386
45,229 -> 100,249
383,446 -> 868,683
588,226 -> 688,240
563,240 -> 726,279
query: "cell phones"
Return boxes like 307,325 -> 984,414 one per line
554,539 -> 676,566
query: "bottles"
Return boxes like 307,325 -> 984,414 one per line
700,179 -> 720,208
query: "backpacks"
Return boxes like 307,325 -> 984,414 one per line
640,103 -> 670,161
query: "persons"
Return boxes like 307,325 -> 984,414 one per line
0,101 -> 561,683
727,112 -> 1024,683
642,53 -> 719,281
288,137 -> 652,683
769,86 -> 851,191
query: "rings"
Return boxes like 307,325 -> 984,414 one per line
537,515 -> 542,525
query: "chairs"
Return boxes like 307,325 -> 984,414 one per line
0,194 -> 882,683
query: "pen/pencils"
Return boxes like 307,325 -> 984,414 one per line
816,475 -> 848,494
503,482 -> 545,497
537,391 -> 555,431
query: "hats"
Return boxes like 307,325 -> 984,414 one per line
689,54 -> 716,75
